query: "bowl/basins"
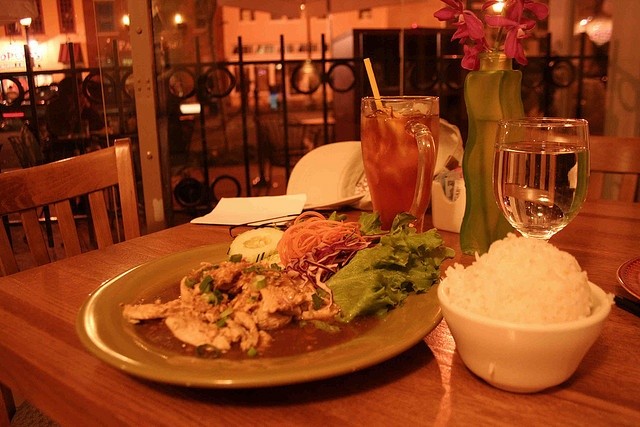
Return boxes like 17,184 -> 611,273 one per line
438,277 -> 613,394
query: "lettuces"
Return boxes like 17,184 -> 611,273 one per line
304,204 -> 455,332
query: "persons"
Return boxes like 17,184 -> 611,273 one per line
42,78 -> 114,164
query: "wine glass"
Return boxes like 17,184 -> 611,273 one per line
493,116 -> 590,241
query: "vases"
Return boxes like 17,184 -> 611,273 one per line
459,54 -> 536,253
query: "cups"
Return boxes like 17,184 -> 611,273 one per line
360,96 -> 441,234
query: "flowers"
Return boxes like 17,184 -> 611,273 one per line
433,0 -> 550,71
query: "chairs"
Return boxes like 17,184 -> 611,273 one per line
583,134 -> 640,203
1,137 -> 141,280
252,110 -> 306,189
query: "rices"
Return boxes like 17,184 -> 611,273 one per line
443,233 -> 595,327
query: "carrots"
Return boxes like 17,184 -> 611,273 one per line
279,208 -> 375,265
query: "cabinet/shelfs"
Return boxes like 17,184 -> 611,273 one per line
330,28 -> 552,141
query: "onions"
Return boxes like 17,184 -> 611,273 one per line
287,239 -> 358,308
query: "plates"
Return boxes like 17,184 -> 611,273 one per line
618,252 -> 640,301
73,241 -> 442,388
